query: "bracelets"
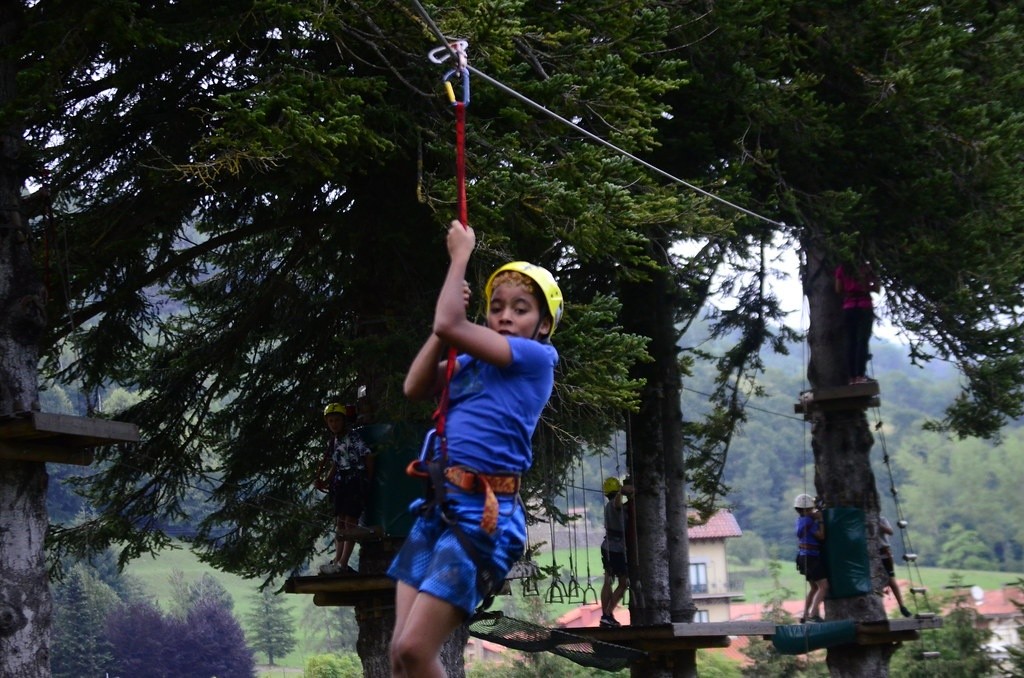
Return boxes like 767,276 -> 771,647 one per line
818,521 -> 824,523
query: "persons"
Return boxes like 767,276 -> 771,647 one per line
878,517 -> 912,618
314,403 -> 374,576
600,476 -> 628,627
388,219 -> 565,678
795,493 -> 827,624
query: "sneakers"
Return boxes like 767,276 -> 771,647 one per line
600,613 -> 620,625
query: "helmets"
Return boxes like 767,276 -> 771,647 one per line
794,494 -> 815,508
604,477 -> 621,495
486,260 -> 565,339
323,403 -> 347,416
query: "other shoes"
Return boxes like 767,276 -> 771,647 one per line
901,606 -> 912,616
318,560 -> 346,574
800,615 -> 824,625
849,375 -> 878,384
